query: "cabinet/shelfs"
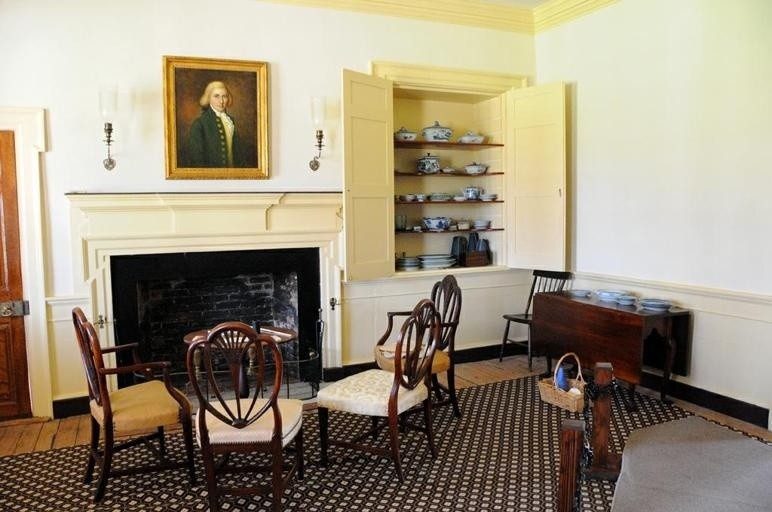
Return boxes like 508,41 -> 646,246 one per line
341,61 -> 567,283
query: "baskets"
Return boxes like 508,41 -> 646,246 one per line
538,353 -> 594,413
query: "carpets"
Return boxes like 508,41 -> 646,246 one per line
0,371 -> 772,512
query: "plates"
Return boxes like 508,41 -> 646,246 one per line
416,253 -> 457,268
479,194 -> 498,201
397,257 -> 420,270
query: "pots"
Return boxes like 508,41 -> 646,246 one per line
421,122 -> 453,143
417,152 -> 440,174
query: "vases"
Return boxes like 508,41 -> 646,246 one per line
451,233 -> 490,266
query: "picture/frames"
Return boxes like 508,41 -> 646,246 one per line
162,55 -> 269,180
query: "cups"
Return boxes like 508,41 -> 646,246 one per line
396,215 -> 407,230
252,319 -> 260,335
449,225 -> 456,231
414,226 -> 421,232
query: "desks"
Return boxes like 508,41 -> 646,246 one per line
533,290 -> 694,411
183,327 -> 297,399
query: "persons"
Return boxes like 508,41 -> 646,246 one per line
187,83 -> 250,168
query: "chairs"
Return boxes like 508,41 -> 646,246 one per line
186,321 -> 304,512
376,275 -> 462,432
72,307 -> 197,503
499,269 -> 572,372
317,299 -> 441,482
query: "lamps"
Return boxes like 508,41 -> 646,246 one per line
99,84 -> 117,170
309,96 -> 328,171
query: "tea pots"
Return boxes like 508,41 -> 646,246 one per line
460,186 -> 482,200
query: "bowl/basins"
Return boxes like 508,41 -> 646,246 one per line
400,195 -> 415,201
459,250 -> 488,267
455,135 -> 485,145
464,165 -> 487,174
570,289 -> 673,312
439,168 -> 456,173
454,194 -> 466,202
427,192 -> 451,202
396,132 -> 417,141
422,216 -> 452,231
415,193 -> 426,202
472,221 -> 489,229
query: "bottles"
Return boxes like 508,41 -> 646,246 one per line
467,232 -> 479,250
452,236 -> 467,261
476,239 -> 487,254
557,368 -> 569,392
